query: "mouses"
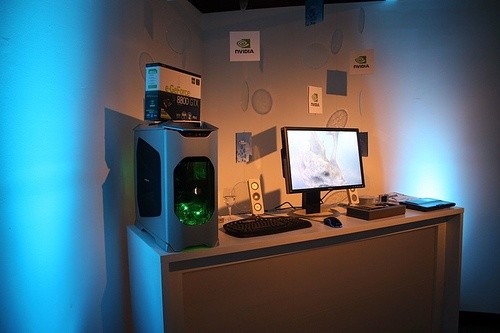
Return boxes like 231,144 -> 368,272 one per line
324,216 -> 342,228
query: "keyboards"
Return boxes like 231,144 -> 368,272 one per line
223,217 -> 312,237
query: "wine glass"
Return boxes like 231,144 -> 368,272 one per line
223,188 -> 237,224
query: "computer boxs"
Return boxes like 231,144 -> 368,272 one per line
135,119 -> 218,254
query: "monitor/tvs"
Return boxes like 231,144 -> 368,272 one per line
281,126 -> 365,218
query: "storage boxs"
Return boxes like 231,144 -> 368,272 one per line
347,202 -> 407,221
144,62 -> 201,122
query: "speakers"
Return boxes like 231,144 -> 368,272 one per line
247,179 -> 264,216
345,188 -> 360,206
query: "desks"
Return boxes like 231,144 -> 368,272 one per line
124,203 -> 464,333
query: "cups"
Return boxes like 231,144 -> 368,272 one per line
359,195 -> 374,206
